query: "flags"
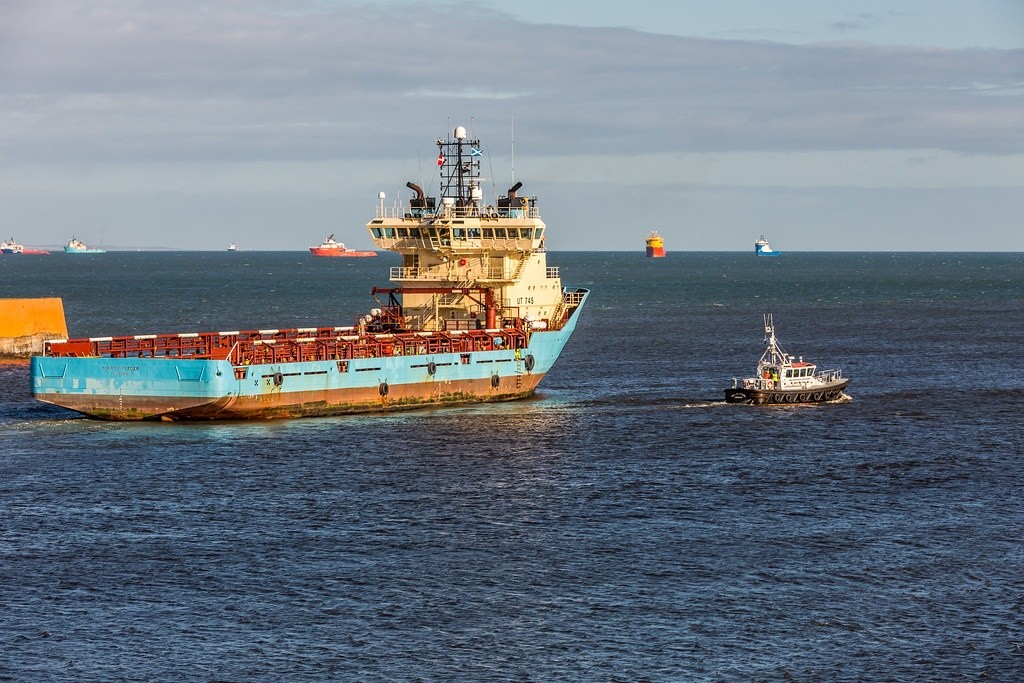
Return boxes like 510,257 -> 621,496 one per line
472,148 -> 483,156
438,153 -> 447,166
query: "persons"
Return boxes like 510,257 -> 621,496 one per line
515,349 -> 520,359
460,231 -> 464,237
140,352 -> 144,358
539,244 -> 542,248
509,231 -> 515,237
523,233 -> 528,239
245,358 -> 252,365
474,229 -> 478,236
393,347 -> 398,355
398,231 -> 401,236
469,229 -> 473,239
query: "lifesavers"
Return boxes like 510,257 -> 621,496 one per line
800,394 -> 805,401
491,375 -> 500,386
774,393 -> 780,403
460,258 -> 466,265
273,373 -> 283,385
814,393 -> 819,400
768,381 -> 773,390
825,392 -> 830,400
525,355 -> 534,371
471,312 -> 476,318
380,383 -> 389,395
428,362 -> 437,374
832,391 -> 836,397
786,394 -> 791,402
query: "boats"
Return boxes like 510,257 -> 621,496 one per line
755,234 -> 782,256
228,243 -> 236,251
63,234 -> 107,253
723,313 -> 853,406
0,237 -> 50,256
309,233 -> 378,258
646,230 -> 667,257
28,112 -> 591,422
